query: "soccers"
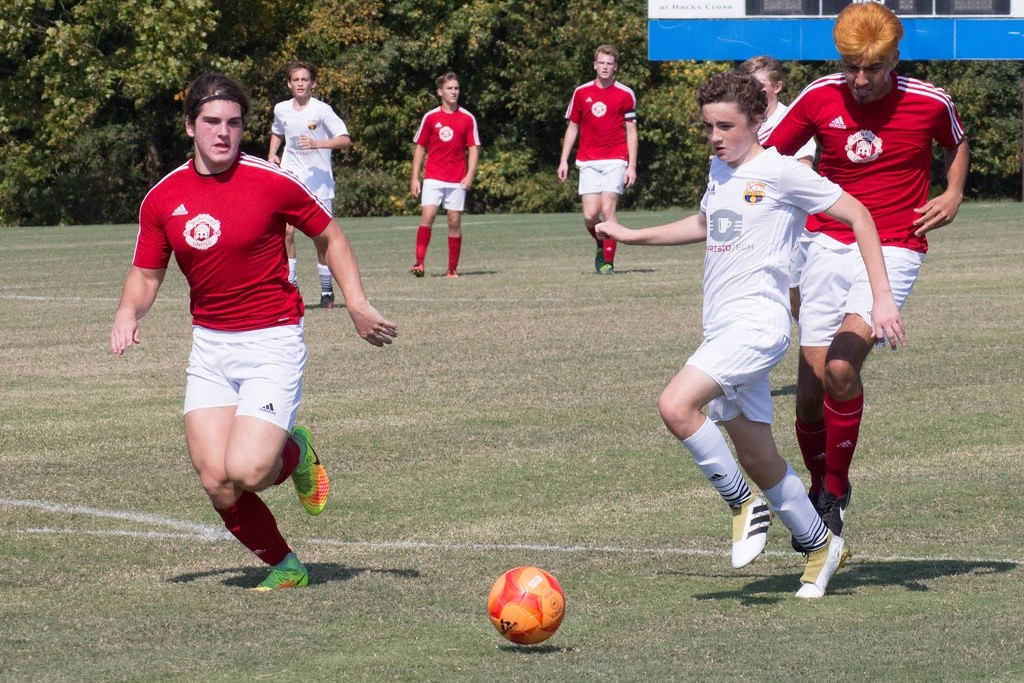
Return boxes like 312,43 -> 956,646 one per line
487,565 -> 566,645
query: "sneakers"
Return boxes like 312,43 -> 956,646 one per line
409,264 -> 424,278
730,495 -> 776,569
791,477 -> 852,553
245,564 -> 309,592
318,293 -> 335,310
289,426 -> 329,515
796,529 -> 853,598
447,270 -> 458,279
594,248 -> 614,274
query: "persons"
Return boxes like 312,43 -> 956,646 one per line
594,74 -> 906,597
268,61 -> 351,309
409,73 -> 481,279
762,3 -> 967,552
739,56 -> 818,321
109,76 -> 398,591
557,44 -> 638,275
